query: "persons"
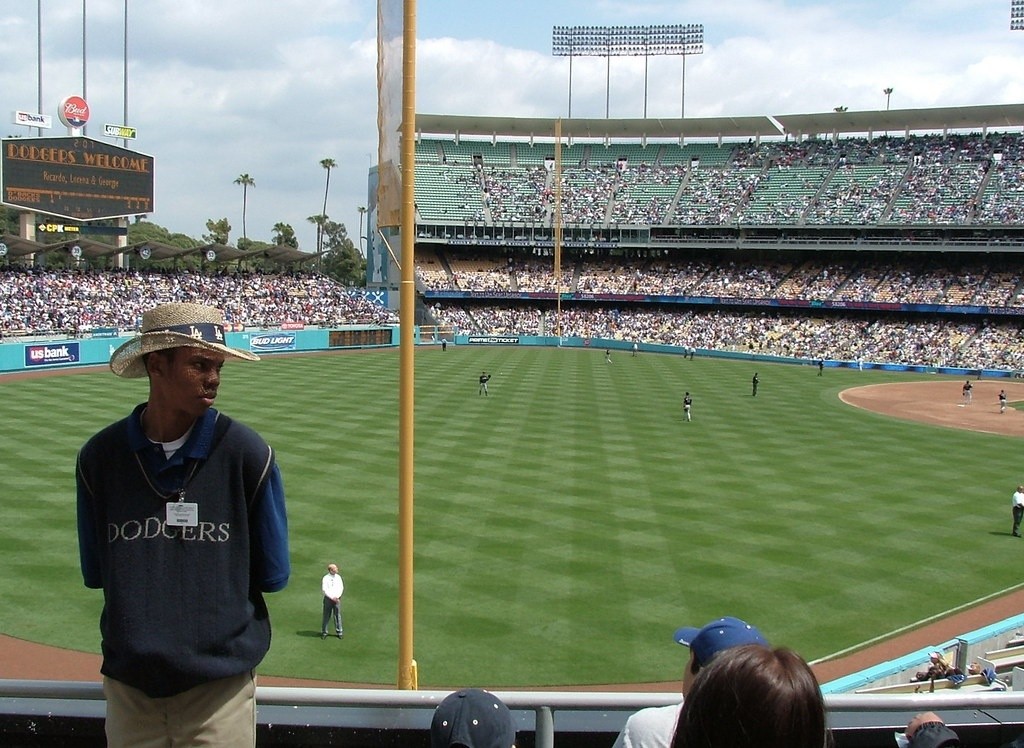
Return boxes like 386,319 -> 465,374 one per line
752,373 -> 760,396
963,381 -> 973,401
430,688 -> 519,748
857,357 -> 863,371
922,652 -> 950,681
1012,485 -> 1024,537
321,564 -> 344,639
683,392 -> 693,422
478,371 -> 489,396
904,710 -> 959,748
818,359 -> 824,376
999,390 -> 1007,413
671,644 -> 826,748
977,363 -> 983,380
74,303 -> 291,748
614,617 -> 772,748
414,138 -> 1024,371
0,261 -> 400,337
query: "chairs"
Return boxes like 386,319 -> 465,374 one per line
414,131 -> 1023,370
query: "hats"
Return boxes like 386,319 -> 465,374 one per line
674,616 -> 767,668
430,688 -> 515,748
935,649 -> 944,656
928,652 -> 937,658
109,303 -> 261,379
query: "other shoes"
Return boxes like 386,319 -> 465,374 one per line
338,635 -> 343,639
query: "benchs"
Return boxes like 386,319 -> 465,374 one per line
854,645 -> 1024,694
